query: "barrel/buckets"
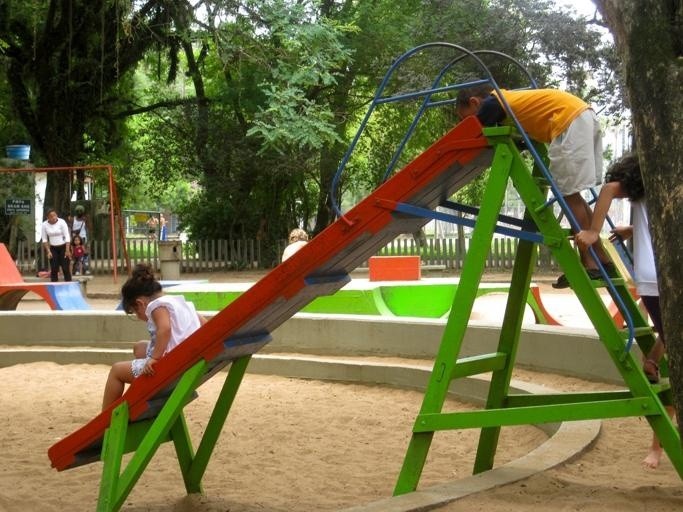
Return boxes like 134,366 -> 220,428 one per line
4,144 -> 31,162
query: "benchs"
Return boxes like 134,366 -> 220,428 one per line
350,265 -> 446,277
22,276 -> 92,297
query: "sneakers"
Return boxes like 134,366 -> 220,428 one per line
74,270 -> 90,275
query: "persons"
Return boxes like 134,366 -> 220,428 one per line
102,262 -> 208,412
639,389 -> 673,471
574,153 -> 665,383
41,204 -> 90,282
281,228 -> 310,262
455,82 -> 617,289
147,213 -> 169,241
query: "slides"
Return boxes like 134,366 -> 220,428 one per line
47,114 -> 495,472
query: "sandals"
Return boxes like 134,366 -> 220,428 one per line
643,358 -> 660,385
552,262 -> 615,288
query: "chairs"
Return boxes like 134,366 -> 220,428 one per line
0,241 -> 91,310
